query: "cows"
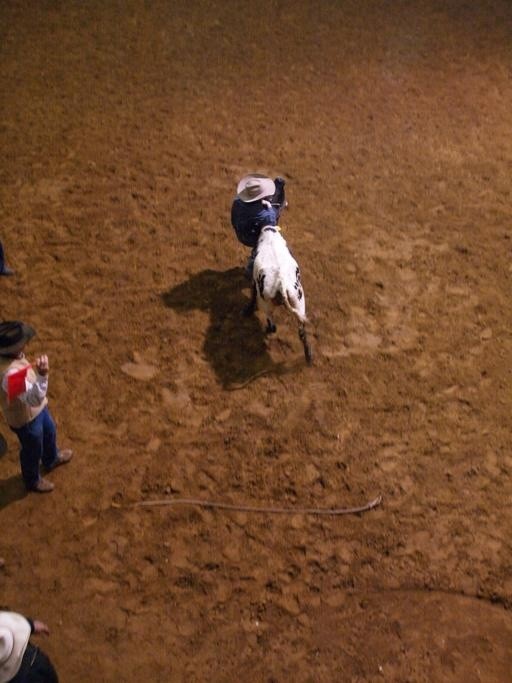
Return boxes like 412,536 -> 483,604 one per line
244,176 -> 313,365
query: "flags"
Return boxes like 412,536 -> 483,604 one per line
8,367 -> 27,404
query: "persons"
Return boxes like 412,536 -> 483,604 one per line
0,321 -> 72,491
231,174 -> 278,280
0,610 -> 59,683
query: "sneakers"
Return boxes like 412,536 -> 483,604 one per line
48,449 -> 73,466
36,478 -> 54,492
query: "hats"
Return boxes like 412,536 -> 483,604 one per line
0,611 -> 32,682
0,319 -> 36,356
237,172 -> 276,203
